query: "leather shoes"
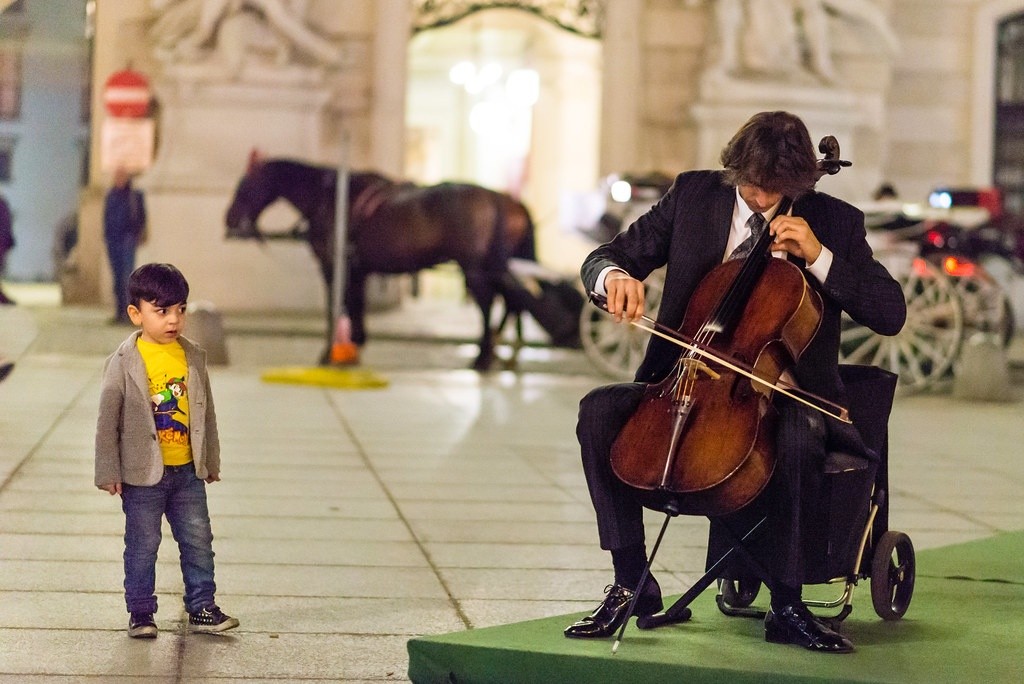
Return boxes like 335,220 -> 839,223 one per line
564,576 -> 665,640
763,599 -> 856,653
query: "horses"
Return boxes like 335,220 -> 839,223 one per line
223,144 -> 540,373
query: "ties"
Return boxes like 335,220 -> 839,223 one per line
727,212 -> 773,262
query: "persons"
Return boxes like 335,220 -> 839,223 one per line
102,169 -> 146,324
563,111 -> 906,652
94,263 -> 240,639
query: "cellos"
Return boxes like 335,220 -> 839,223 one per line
609,133 -> 852,654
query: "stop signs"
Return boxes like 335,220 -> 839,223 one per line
101,72 -> 152,117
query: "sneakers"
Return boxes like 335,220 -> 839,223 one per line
126,611 -> 158,637
185,603 -> 240,634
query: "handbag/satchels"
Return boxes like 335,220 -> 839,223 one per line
132,191 -> 152,249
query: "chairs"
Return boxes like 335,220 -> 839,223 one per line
706,363 -> 917,621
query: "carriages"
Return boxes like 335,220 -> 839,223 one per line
225,147 -> 1017,396
